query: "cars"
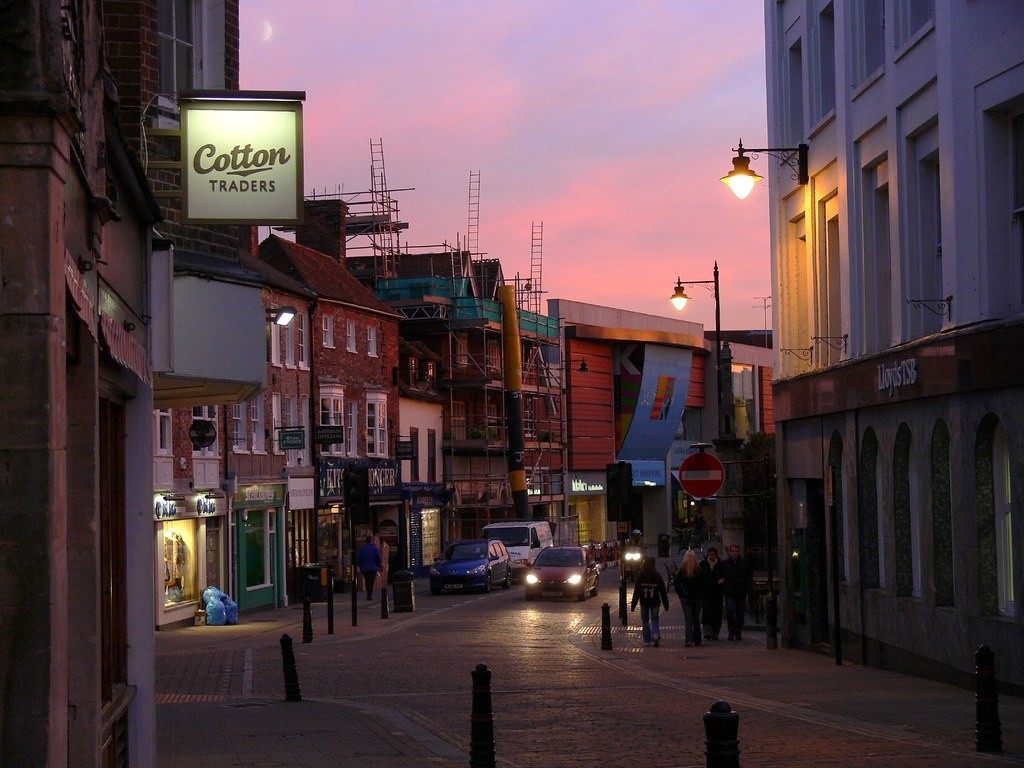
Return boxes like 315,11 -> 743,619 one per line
524,545 -> 601,601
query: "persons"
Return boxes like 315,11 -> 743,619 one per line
631,557 -> 669,647
673,544 -> 750,647
695,510 -> 705,543
355,536 -> 382,600
628,529 -> 646,556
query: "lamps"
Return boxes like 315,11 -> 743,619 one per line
562,358 -> 588,372
719,137 -> 809,200
163,494 -> 185,501
205,493 -> 224,499
265,306 -> 297,326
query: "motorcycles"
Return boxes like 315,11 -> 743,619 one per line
622,538 -> 648,581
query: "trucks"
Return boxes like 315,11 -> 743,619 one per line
476,521 -> 555,583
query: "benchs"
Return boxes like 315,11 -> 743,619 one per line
663,560 -> 678,593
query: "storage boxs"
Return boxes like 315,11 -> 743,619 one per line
194,611 -> 206,626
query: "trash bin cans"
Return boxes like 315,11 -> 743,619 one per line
657,534 -> 670,558
391,570 -> 416,613
301,563 -> 334,603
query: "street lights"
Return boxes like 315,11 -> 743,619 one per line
669,259 -> 723,437
556,348 -> 591,517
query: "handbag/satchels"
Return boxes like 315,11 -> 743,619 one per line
674,570 -> 688,595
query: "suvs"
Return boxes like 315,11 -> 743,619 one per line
428,537 -> 513,594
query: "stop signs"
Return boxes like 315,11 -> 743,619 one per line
680,452 -> 726,498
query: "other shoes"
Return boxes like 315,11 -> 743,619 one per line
653,635 -> 660,648
705,636 -> 719,642
695,638 -> 702,646
685,641 -> 693,648
736,637 -> 742,641
727,634 -> 733,640
643,639 -> 649,646
